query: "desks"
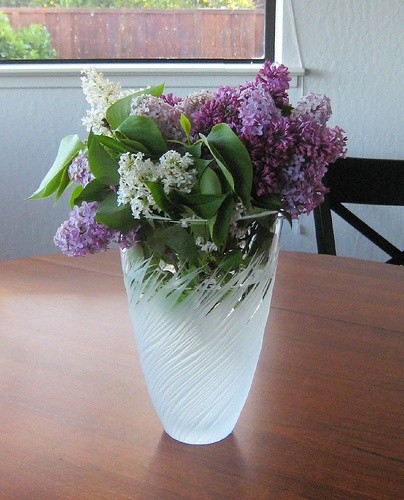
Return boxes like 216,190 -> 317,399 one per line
0,250 -> 403,496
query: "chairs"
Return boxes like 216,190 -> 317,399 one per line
312,158 -> 404,265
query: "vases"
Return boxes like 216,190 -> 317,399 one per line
119,206 -> 285,444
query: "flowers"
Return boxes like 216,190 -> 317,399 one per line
25,59 -> 349,258
